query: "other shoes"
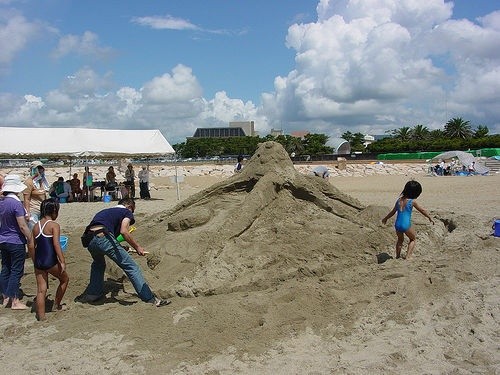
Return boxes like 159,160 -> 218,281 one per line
152,300 -> 171,307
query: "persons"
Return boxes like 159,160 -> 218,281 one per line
314,172 -> 330,178
427,158 -> 476,176
236,161 -> 242,171
382,180 -> 435,259
0,160 -> 172,321
290,151 -> 296,164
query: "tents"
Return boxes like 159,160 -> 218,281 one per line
0,126 -> 180,202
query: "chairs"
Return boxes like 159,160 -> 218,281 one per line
104,182 -> 118,200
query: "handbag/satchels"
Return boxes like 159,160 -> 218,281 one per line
81,228 -> 106,248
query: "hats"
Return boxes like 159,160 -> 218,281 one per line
127,164 -> 134,168
0,175 -> 28,193
